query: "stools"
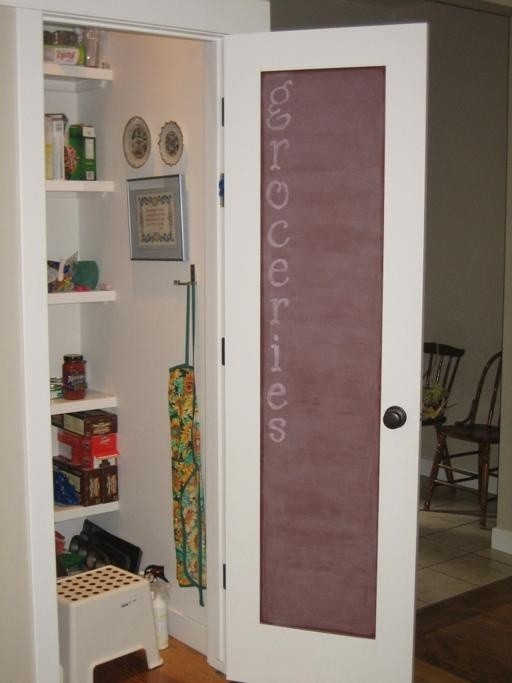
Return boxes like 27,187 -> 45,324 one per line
53,567 -> 164,680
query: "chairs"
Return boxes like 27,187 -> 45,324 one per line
426,350 -> 504,530
420,342 -> 464,511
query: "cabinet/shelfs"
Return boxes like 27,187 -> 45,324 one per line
0,0 -> 431,683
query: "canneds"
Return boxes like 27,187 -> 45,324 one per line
50,377 -> 64,385
62,353 -> 87,400
49,384 -> 63,400
